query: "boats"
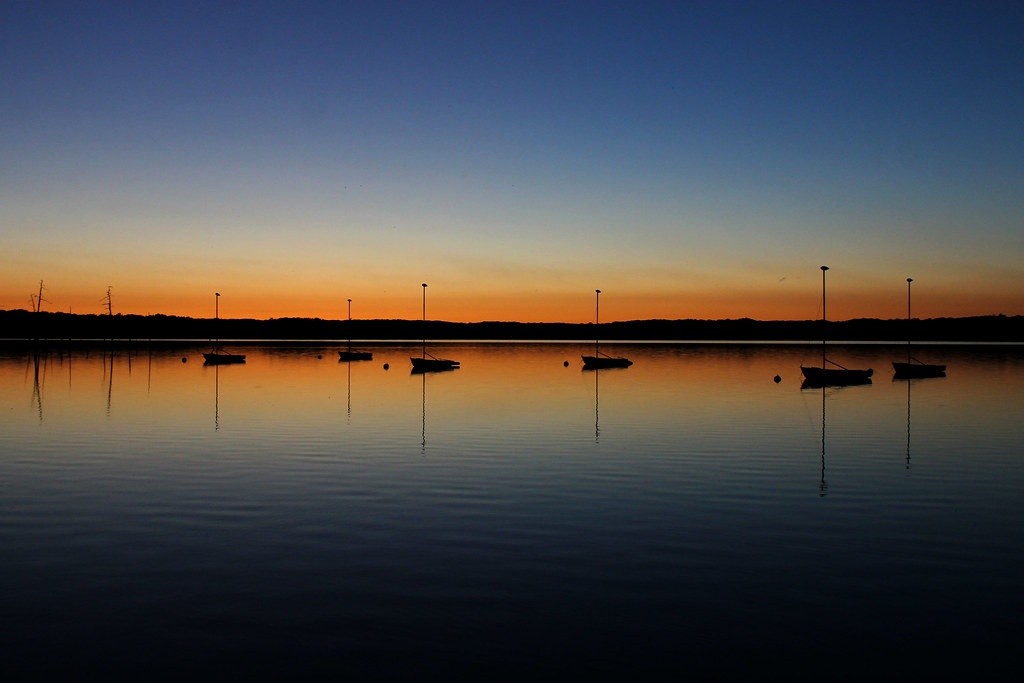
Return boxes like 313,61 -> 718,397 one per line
581,290 -> 633,367
202,293 -> 246,364
410,283 -> 460,370
800,266 -> 873,383
338,299 -> 373,360
892,278 -> 947,377
